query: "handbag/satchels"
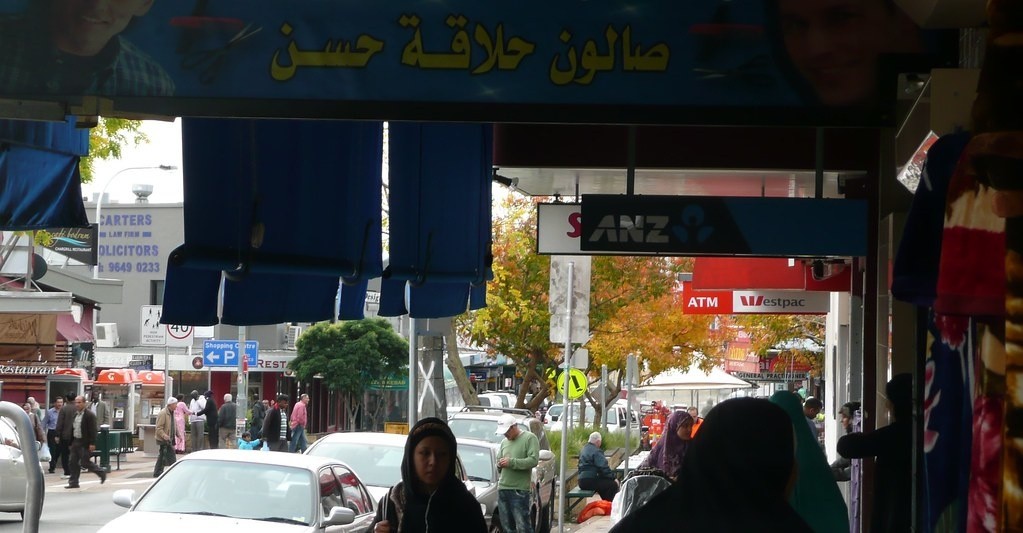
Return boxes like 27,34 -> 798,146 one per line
260,442 -> 269,451
160,441 -> 176,466
38,442 -> 51,462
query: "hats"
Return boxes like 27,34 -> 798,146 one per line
495,415 -> 517,435
803,396 -> 822,409
887,374 -> 913,405
168,397 -> 178,404
839,402 -> 861,418
204,391 -> 213,397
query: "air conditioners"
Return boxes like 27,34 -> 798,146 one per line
288,326 -> 302,350
96,322 -> 119,347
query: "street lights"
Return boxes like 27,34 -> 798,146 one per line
93,165 -> 179,342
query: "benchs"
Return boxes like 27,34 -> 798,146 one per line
564,486 -> 597,515
616,448 -> 650,480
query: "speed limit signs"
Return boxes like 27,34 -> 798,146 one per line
166,323 -> 192,339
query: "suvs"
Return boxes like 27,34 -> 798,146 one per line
443,406 -> 557,533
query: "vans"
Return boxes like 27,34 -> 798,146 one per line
543,398 -> 641,458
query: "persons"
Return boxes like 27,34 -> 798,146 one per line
23,391 -> 107,488
837,372 -> 912,533
86,391 -> 109,432
153,397 -> 178,477
830,401 -> 861,481
289,394 -> 309,454
258,394 -> 292,452
577,431 -> 620,502
803,398 -> 822,442
218,394 -> 236,449
249,393 -> 270,441
609,397 -> 817,533
172,390 -> 218,454
238,431 -> 263,450
365,417 -> 488,533
701,400 -> 713,419
769,391 -> 850,533
495,414 -> 540,533
638,411 -> 693,482
687,407 -> 703,438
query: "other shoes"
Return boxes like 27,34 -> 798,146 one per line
154,474 -> 159,477
48,470 -> 53,473
81,468 -> 90,473
61,474 -> 71,480
98,468 -> 107,484
64,482 -> 79,488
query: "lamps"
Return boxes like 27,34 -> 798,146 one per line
492,167 -> 520,192
903,70 -> 926,96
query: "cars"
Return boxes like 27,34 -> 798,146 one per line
457,389 -> 549,411
95,448 -> 381,533
0,415 -> 44,518
301,432 -> 502,533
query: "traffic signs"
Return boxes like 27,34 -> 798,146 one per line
203,339 -> 259,367
